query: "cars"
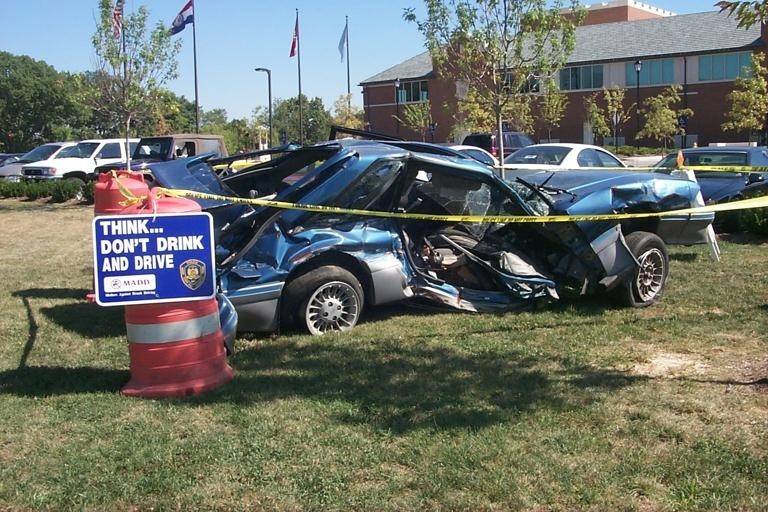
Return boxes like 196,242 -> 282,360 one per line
415,143 -> 500,181
649,146 -> 768,235
0,141 -> 78,183
143,125 -> 716,355
493,142 -> 635,172
212,159 -> 261,176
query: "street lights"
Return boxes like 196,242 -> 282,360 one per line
634,60 -> 642,150
613,110 -> 621,154
394,78 -> 401,138
254,67 -> 272,161
429,119 -> 438,142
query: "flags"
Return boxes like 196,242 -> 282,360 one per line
112,0 -> 125,39
290,12 -> 299,57
169,0 -> 194,36
338,24 -> 348,63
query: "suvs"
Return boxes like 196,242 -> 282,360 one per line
18,138 -> 152,201
461,132 -> 535,161
94,133 -> 230,187
